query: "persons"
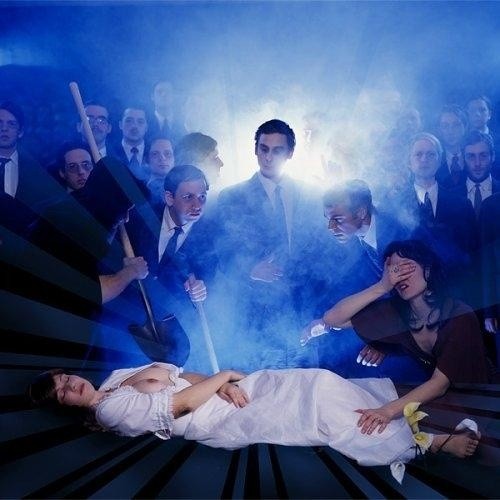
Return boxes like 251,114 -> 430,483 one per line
1,79 -> 498,384
29,361 -> 481,467
323,241 -> 489,434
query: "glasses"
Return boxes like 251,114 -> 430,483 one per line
0,120 -> 22,132
86,116 -> 112,127
65,161 -> 93,173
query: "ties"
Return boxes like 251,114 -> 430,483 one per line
360,240 -> 384,273
474,184 -> 482,221
130,148 -> 140,171
0,157 -> 12,192
450,155 -> 462,177
158,225 -> 184,277
424,192 -> 435,223
161,119 -> 173,138
274,186 -> 290,260
